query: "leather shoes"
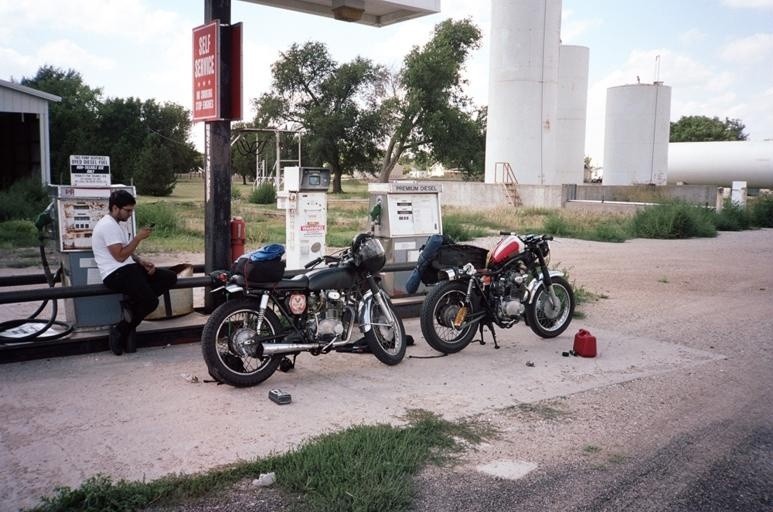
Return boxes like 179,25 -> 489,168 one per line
119,300 -> 134,324
109,323 -> 124,355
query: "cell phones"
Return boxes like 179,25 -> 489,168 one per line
150,223 -> 155,227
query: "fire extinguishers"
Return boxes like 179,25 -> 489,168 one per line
231,216 -> 245,262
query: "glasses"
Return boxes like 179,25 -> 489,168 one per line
122,208 -> 131,212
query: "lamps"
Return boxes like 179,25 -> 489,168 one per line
331,0 -> 366,21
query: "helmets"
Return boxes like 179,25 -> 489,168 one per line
355,239 -> 385,274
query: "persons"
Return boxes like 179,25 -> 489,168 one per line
91,189 -> 179,356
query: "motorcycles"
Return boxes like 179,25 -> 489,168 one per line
419,231 -> 574,355
200,201 -> 408,388
